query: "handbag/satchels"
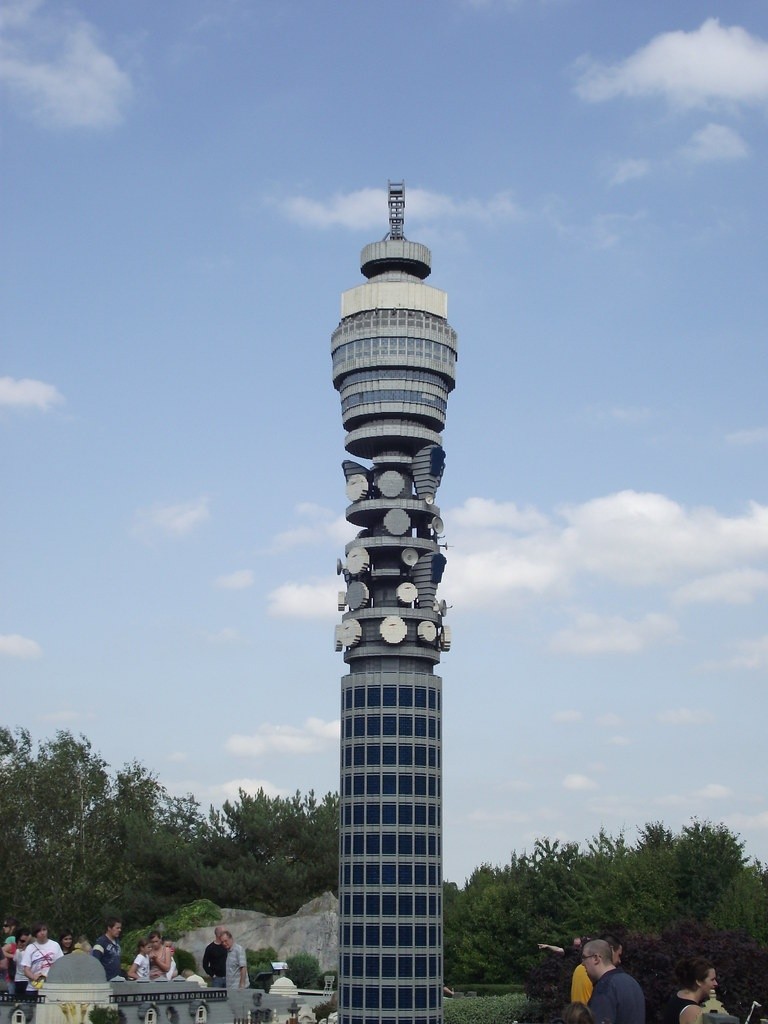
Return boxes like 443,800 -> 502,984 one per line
8,959 -> 16,980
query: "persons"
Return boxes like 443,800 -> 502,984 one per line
566,1002 -> 595,1024
127,930 -> 207,987
220,931 -> 250,989
93,917 -> 123,981
664,958 -> 718,1024
203,927 -> 227,988
524,937 -> 590,1024
443,979 -> 454,998
582,939 -> 645,1024
570,934 -> 623,1006
0,918 -> 108,998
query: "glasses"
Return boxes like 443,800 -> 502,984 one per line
582,955 -> 602,961
19,939 -> 25,943
3,924 -> 9,927
